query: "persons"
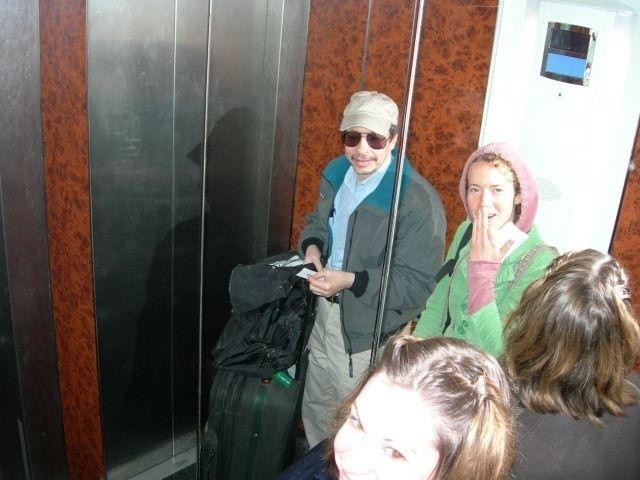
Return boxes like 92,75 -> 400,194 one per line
276,335 -> 516,480
497,249 -> 640,479
296,89 -> 447,447
411,139 -> 561,360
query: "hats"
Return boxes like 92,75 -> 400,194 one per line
340,91 -> 400,139
460,143 -> 536,234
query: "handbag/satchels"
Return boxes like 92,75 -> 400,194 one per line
211,251 -> 317,379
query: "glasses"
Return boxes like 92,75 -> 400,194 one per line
341,132 -> 390,149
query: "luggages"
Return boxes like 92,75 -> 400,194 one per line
200,256 -> 328,480
